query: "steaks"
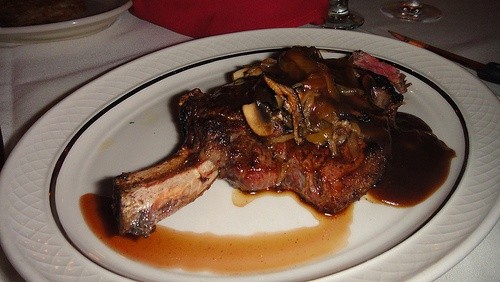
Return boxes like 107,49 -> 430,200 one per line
107,46 -> 414,238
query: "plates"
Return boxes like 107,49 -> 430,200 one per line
0,27 -> 500,282
0,0 -> 133,42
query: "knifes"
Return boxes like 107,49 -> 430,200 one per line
386,29 -> 500,86
